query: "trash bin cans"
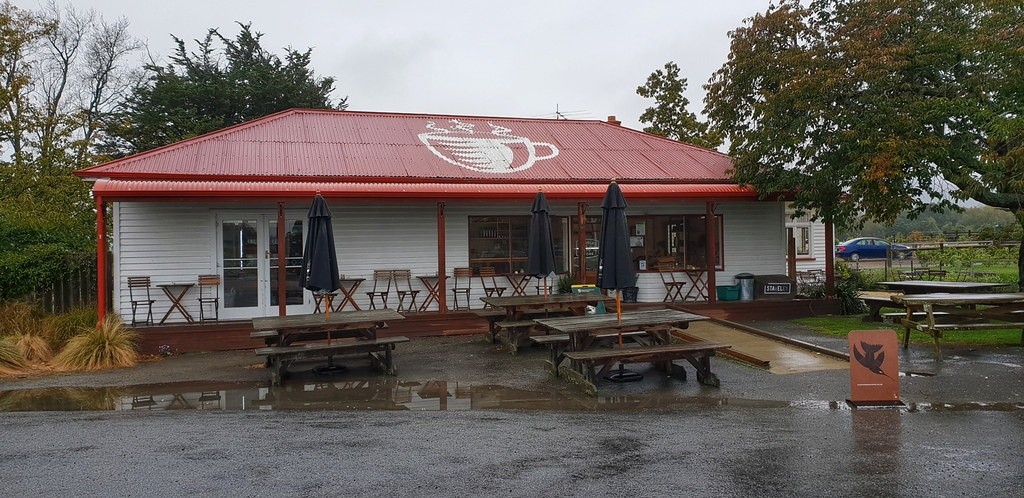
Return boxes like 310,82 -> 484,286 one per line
735,272 -> 754,300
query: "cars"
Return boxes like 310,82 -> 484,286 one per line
835,236 -> 913,263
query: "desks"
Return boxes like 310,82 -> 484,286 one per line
889,293 -> 1024,362
504,273 -> 539,296
334,278 -> 366,311
862,281 -> 1010,322
533,309 -> 710,397
251,309 -> 406,386
416,276 -> 453,312
479,292 -> 614,356
682,269 -> 721,303
155,284 -> 195,325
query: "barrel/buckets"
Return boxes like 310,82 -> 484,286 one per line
734,272 -> 755,301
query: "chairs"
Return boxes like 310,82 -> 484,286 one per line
127,274 -> 221,327
479,266 -> 507,310
308,277 -> 337,314
366,269 -> 421,314
451,267 -> 473,310
658,265 -> 686,303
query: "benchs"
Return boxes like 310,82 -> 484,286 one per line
854,296 -> 1024,361
474,308 -> 732,398
248,323 -> 411,386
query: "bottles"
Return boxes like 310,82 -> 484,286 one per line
515,267 -> 524,274
436,270 -> 438,276
341,274 -> 345,279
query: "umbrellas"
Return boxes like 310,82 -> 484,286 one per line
299,188 -> 340,368
595,176 -> 635,374
525,187 -> 555,335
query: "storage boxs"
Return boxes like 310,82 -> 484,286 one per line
716,285 -> 739,300
